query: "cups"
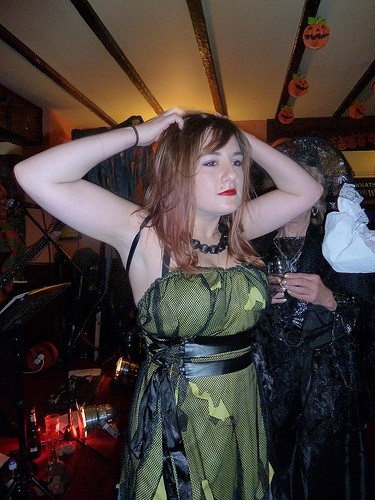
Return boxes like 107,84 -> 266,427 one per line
45,413 -> 59,464
268,260 -> 293,291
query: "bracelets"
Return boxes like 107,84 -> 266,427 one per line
131,124 -> 139,147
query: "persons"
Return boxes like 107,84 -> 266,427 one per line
13,108 -> 324,500
235,139 -> 375,500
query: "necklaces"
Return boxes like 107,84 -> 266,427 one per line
179,219 -> 229,256
274,211 -> 311,263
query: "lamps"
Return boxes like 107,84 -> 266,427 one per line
80,403 -> 122,441
113,356 -> 142,384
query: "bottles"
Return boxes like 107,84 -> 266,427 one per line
28,421 -> 42,459
45,447 -> 66,499
7,458 -> 24,500
